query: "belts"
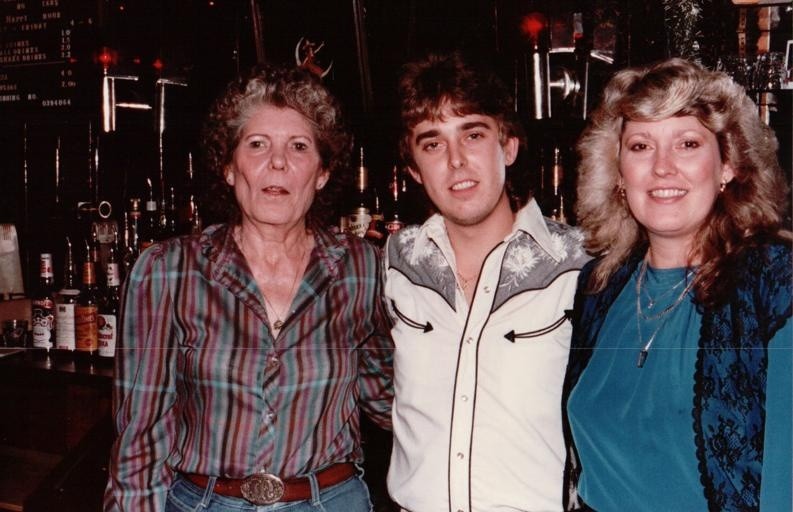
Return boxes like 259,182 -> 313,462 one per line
178,461 -> 365,507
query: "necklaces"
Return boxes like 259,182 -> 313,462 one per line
259,251 -> 306,329
636,243 -> 703,368
457,271 -> 477,290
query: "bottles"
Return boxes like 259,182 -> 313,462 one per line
330,145 -> 422,251
4,188 -> 206,369
727,50 -> 789,130
544,140 -> 571,227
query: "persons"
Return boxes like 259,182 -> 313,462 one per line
560,56 -> 793,512
102,63 -> 395,511
